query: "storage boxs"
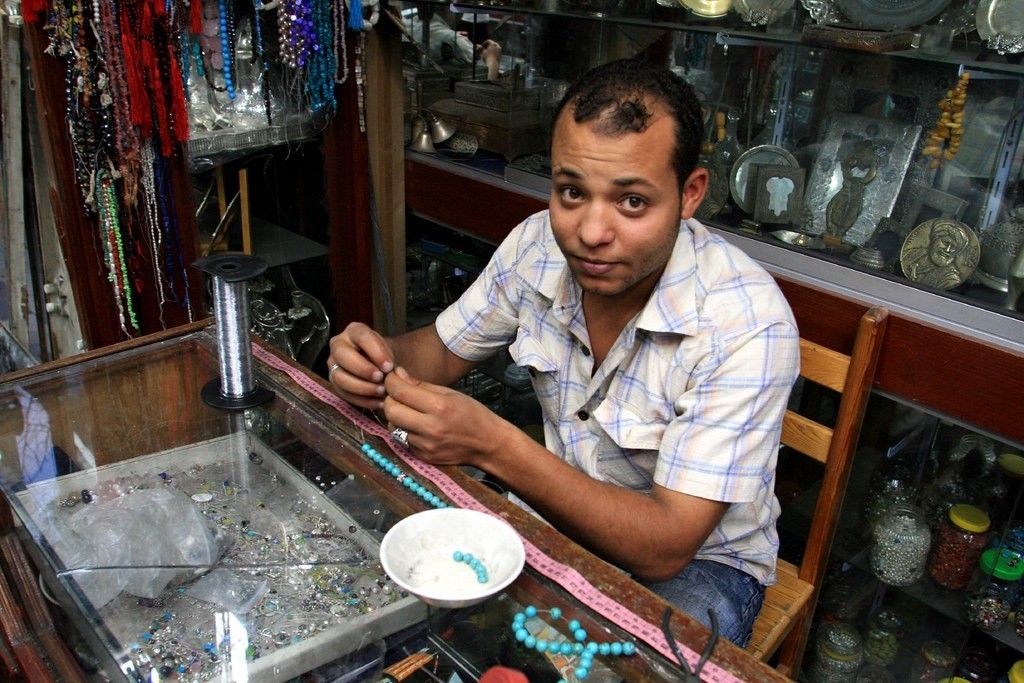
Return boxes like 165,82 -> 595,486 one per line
8,430 -> 428,683
425,75 -> 572,163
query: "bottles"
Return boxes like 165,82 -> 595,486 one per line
810,460 -> 1024,683
711,110 -> 742,217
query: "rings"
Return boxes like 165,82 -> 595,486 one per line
330,363 -> 339,372
391,427 -> 410,448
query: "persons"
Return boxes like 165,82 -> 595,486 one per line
324,55 -> 801,649
402,0 -> 502,80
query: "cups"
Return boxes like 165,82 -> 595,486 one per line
918,24 -> 956,61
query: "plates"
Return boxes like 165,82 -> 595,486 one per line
679,1 -> 1024,55
695,155 -> 728,223
729,144 -> 800,214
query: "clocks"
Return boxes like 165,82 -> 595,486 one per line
835,0 -> 951,30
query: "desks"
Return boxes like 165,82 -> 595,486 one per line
0,315 -> 803,683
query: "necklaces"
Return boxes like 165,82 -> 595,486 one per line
511,606 -> 635,683
359,441 -> 454,510
22,0 -> 380,341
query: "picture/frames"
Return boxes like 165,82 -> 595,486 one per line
792,110 -> 924,248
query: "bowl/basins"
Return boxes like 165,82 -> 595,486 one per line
379,508 -> 526,610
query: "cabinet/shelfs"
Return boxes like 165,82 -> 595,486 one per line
403,0 -> 1024,651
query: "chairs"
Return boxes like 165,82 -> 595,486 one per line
739,305 -> 890,683
